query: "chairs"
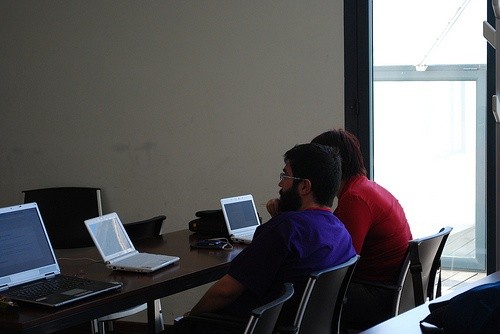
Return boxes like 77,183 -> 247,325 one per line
158,254 -> 361,334
347,228 -> 451,334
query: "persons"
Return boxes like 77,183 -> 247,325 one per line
173,142 -> 355,334
312,129 -> 413,334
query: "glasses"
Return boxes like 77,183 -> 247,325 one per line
279,172 -> 303,181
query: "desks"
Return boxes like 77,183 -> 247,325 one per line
360,271 -> 500,334
0,226 -> 253,334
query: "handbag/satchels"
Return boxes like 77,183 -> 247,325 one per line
420,281 -> 500,334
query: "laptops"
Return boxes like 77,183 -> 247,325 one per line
84,212 -> 180,273
0,202 -> 123,310
220,194 -> 261,244
